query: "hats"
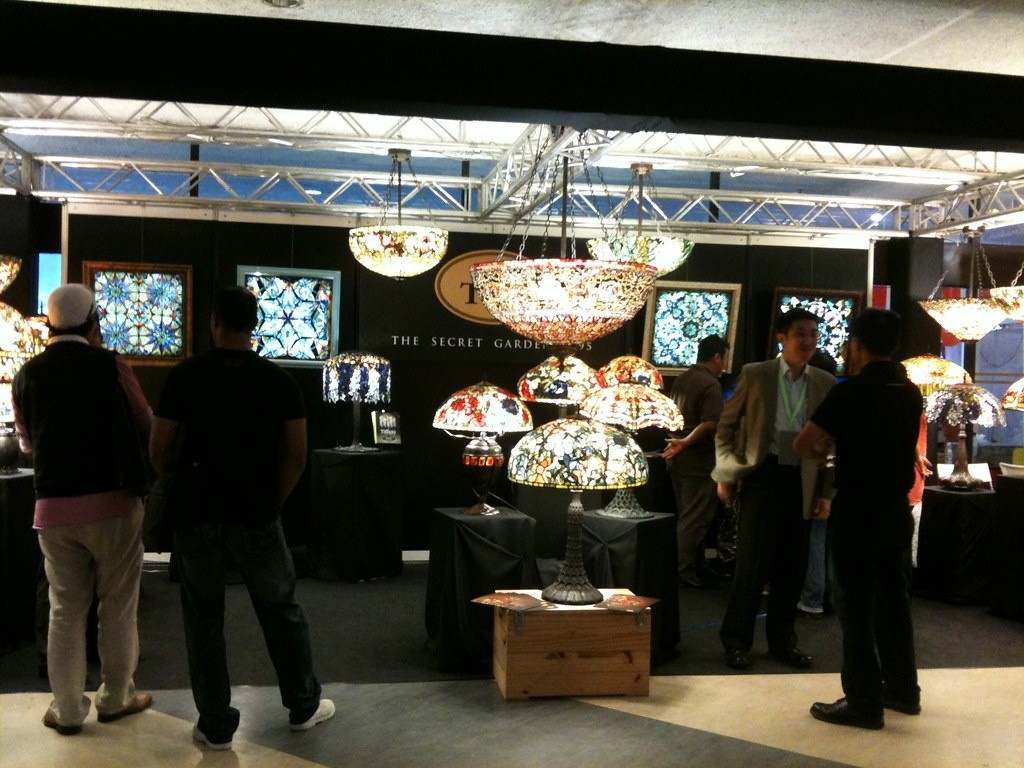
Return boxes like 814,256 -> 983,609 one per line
47,284 -> 95,329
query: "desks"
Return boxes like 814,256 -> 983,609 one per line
306,448 -> 408,584
917,484 -> 1000,603
0,467 -> 41,655
511,489 -> 602,556
584,507 -> 681,652
989,473 -> 1024,627
425,504 -> 540,671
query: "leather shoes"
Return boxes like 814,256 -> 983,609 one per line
96,691 -> 152,723
42,709 -> 82,736
880,679 -> 922,715
809,693 -> 885,731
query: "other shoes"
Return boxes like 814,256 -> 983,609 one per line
727,647 -> 749,669
766,646 -> 813,667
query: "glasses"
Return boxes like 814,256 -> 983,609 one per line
787,327 -> 821,340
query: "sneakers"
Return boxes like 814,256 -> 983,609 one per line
290,699 -> 336,730
191,721 -> 233,749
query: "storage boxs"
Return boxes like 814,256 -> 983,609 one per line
487,589 -> 654,704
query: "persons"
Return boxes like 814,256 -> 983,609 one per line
13,282 -> 151,735
841,345 -> 934,569
793,308 -> 923,730
710,308 -> 837,670
660,335 -> 731,586
148,286 -> 337,749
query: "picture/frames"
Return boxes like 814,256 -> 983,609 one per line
767,286 -> 865,379
642,279 -> 744,379
83,261 -> 196,369
235,264 -> 343,370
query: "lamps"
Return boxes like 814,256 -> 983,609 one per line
919,228 -> 1009,343
517,351 -> 595,417
0,255 -> 48,477
901,351 -> 973,397
317,350 -> 392,452
922,380 -> 1009,492
1001,376 -> 1024,412
507,418 -> 651,603
577,381 -> 686,518
470,123 -> 658,348
990,264 -> 1024,321
347,148 -> 449,281
586,162 -> 695,277
596,352 -> 666,391
430,378 -> 536,518
